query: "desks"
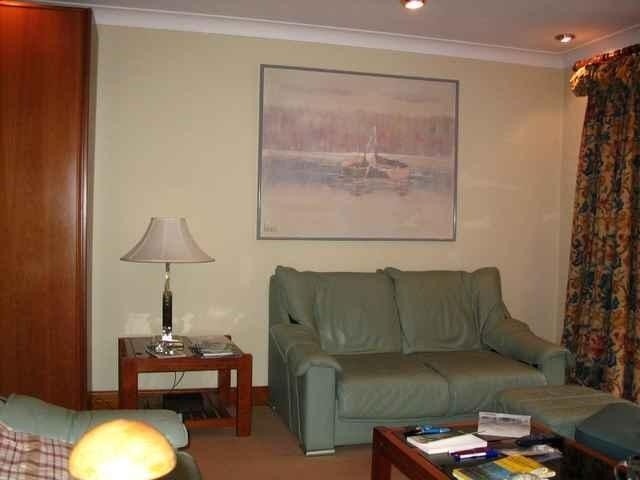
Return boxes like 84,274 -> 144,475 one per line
119,337 -> 252,438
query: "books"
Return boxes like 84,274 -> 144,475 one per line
452,453 -> 556,480
407,429 -> 489,456
477,411 -> 532,438
193,335 -> 241,359
146,348 -> 188,358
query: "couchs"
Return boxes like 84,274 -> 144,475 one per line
0,392 -> 199,480
269,266 -> 576,457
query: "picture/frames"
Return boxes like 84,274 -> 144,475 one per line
256,63 -> 459,242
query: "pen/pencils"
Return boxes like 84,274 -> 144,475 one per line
448,447 -> 498,461
404,427 -> 451,438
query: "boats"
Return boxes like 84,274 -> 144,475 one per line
338,121 -> 415,181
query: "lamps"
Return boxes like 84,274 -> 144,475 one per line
120,218 -> 216,350
68,419 -> 178,479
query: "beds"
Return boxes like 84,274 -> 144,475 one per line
495,385 -> 639,479
